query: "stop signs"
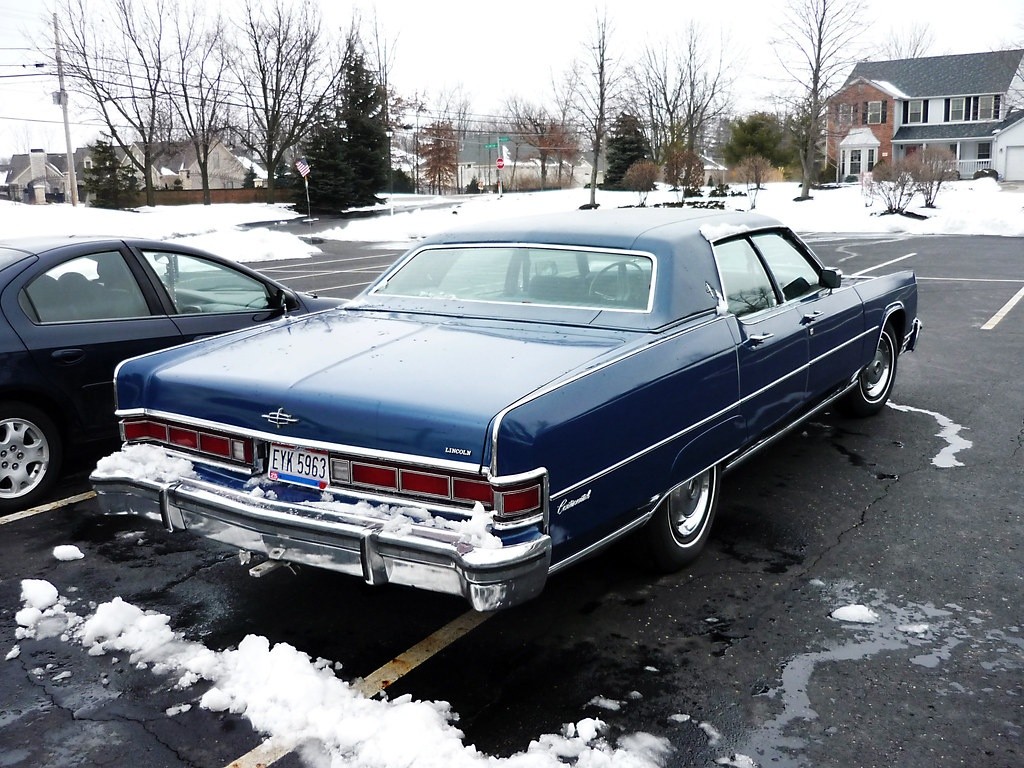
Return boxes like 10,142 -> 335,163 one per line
496,158 -> 504,169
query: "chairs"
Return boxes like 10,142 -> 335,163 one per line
97,256 -> 149,318
30,275 -> 66,322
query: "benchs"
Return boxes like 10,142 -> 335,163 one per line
530,275 -> 650,309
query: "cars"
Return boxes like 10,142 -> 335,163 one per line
1,234 -> 356,517
88,208 -> 925,615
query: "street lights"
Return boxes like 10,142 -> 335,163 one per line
386,126 -> 394,217
